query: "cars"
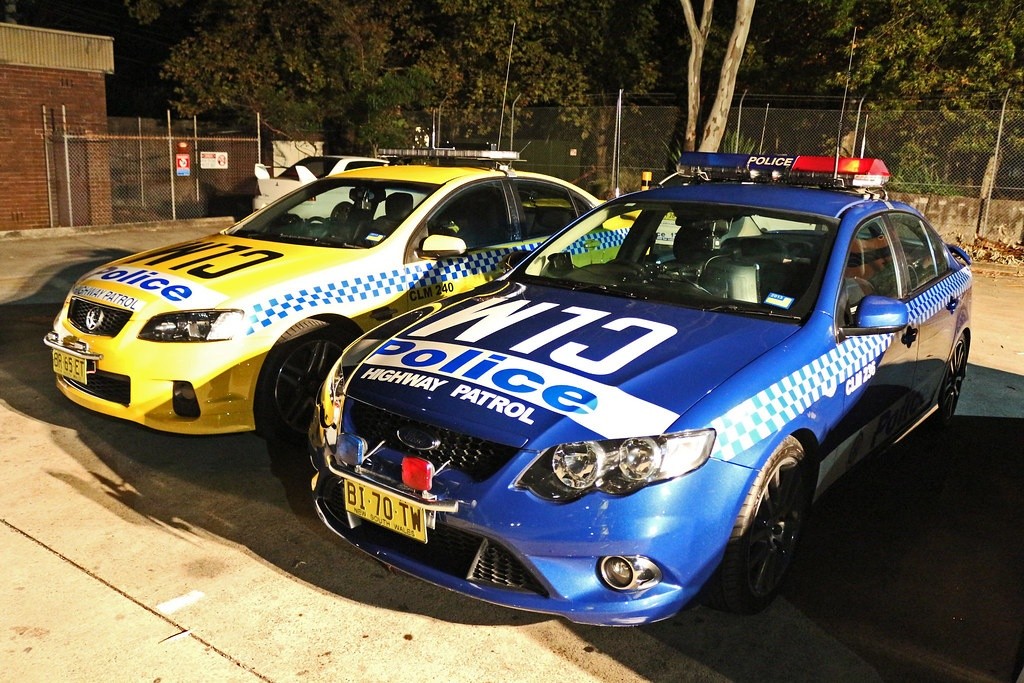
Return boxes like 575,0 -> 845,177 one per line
251,154 -> 430,233
40,146 -> 656,456
301,150 -> 973,636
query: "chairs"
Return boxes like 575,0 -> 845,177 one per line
660,225 -> 714,284
844,236 -> 873,306
860,236 -> 892,272
531,206 -> 572,237
373,192 -> 412,236
332,187 -> 359,226
